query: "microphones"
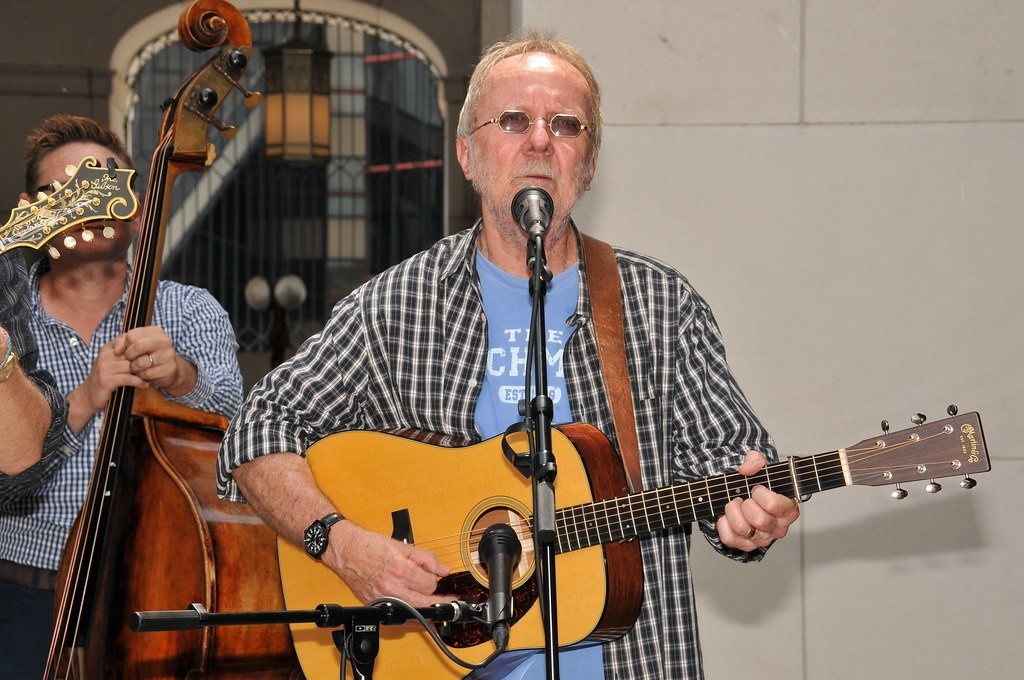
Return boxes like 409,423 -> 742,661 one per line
512,187 -> 554,239
477,522 -> 522,651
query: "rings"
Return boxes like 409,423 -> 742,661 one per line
741,526 -> 756,539
148,352 -> 153,367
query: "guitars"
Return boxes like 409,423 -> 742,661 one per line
276,405 -> 991,680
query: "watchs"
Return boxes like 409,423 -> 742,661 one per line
302,512 -> 345,561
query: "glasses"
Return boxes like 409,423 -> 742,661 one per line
462,110 -> 597,146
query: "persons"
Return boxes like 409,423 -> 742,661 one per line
0,224 -> 70,518
211,23 -> 800,678
0,114 -> 242,680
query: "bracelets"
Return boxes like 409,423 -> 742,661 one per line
0,344 -> 20,383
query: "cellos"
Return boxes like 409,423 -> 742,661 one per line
41,2 -> 308,680
